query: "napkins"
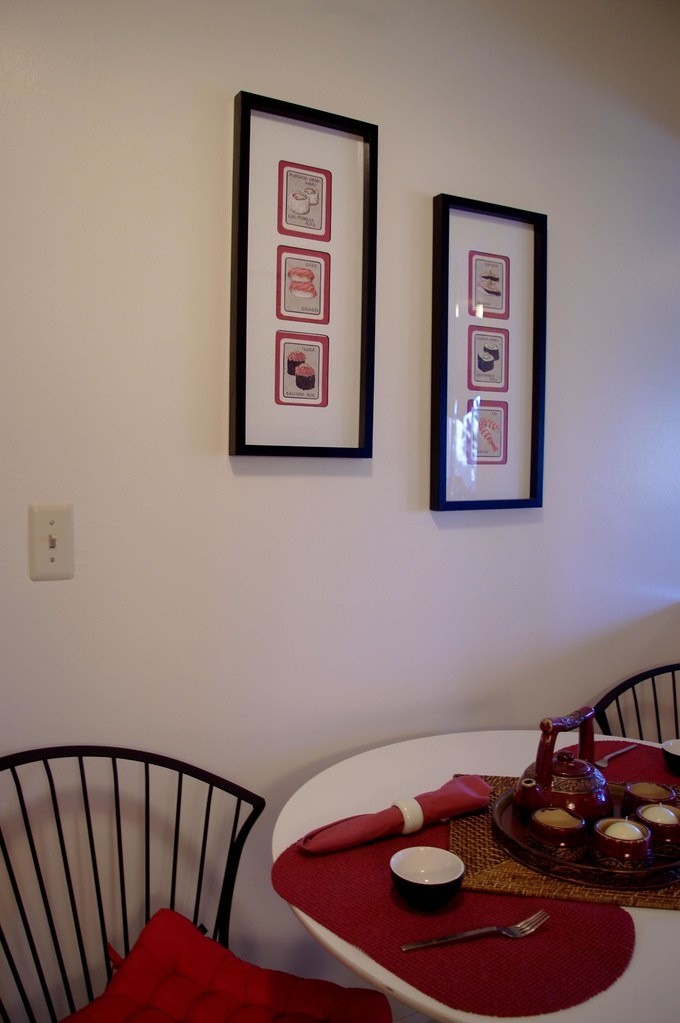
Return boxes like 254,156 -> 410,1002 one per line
294,772 -> 492,857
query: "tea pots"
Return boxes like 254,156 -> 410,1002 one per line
513,704 -> 614,834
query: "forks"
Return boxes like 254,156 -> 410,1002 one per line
591,744 -> 637,768
399,909 -> 550,955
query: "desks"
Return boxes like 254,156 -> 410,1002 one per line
273,730 -> 680,1023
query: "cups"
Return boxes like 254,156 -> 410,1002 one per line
661,738 -> 680,773
590,816 -> 654,870
524,803 -> 589,863
619,781 -> 676,819
634,802 -> 680,861
390,847 -> 466,910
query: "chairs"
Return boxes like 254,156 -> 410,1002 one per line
0,742 -> 268,1023
594,663 -> 680,744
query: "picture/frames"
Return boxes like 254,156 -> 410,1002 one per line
426,191 -> 549,513
229,90 -> 379,458
